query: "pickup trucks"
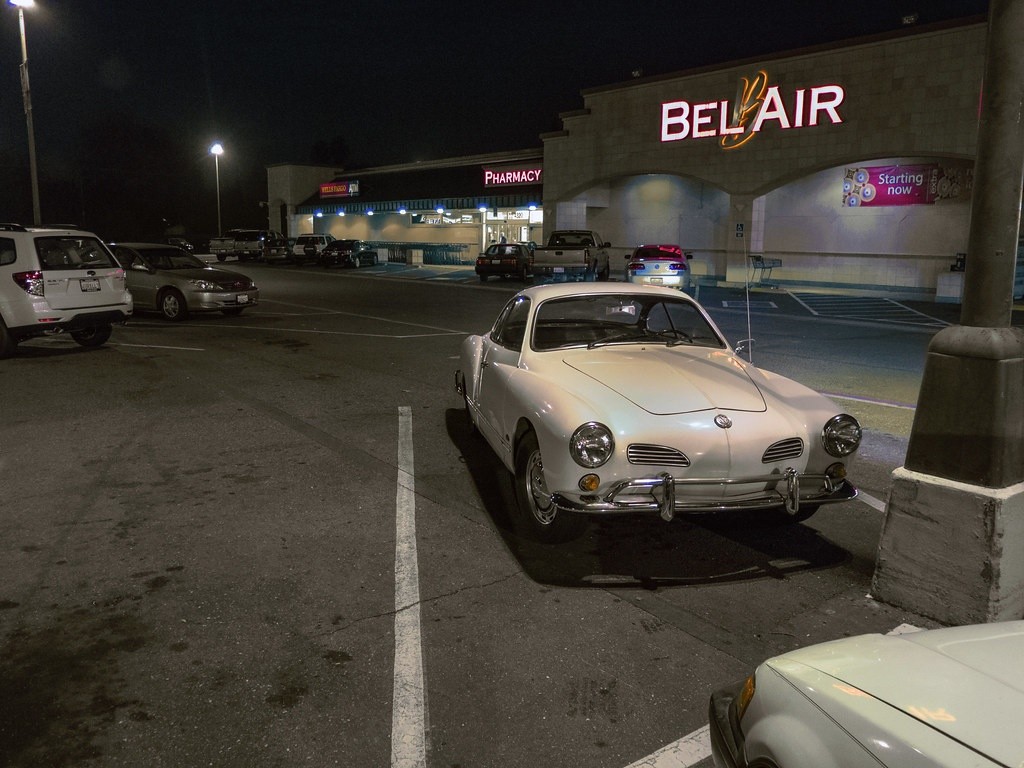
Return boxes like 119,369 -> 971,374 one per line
528,229 -> 613,283
209,229 -> 243,262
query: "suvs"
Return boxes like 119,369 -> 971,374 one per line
0,221 -> 136,359
234,229 -> 288,262
291,232 -> 337,263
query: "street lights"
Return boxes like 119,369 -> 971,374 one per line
4,0 -> 44,226
209,142 -> 223,237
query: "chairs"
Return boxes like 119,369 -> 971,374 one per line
556,238 -> 566,246
497,249 -> 505,255
0,249 -> 16,265
46,249 -> 64,265
581,238 -> 592,245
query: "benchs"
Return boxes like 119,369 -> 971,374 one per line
505,326 -> 645,344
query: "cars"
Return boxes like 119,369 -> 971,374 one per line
623,243 -> 695,293
320,238 -> 379,269
455,281 -> 867,544
475,242 -> 534,281
75,241 -> 260,323
706,615 -> 1023,767
